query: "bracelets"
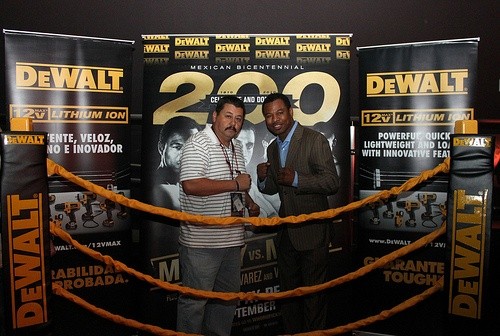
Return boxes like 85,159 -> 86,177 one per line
235,179 -> 240,191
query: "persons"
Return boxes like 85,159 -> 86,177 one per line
148,116 -> 337,251
256,94 -> 341,333
175,96 -> 260,334
